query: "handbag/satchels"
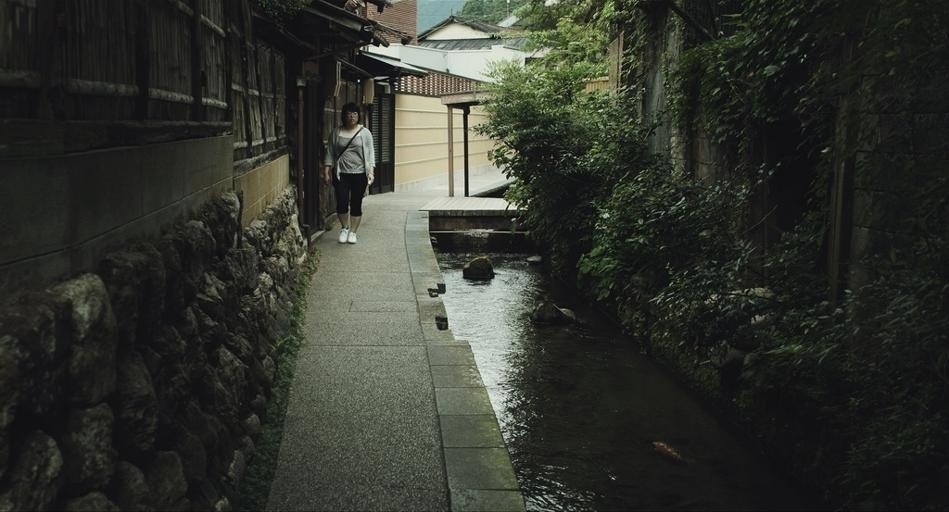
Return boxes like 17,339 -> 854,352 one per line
330,164 -> 340,188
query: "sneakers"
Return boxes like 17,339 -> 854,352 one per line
337,226 -> 350,243
347,231 -> 357,243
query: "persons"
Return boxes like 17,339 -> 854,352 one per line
322,101 -> 376,244
